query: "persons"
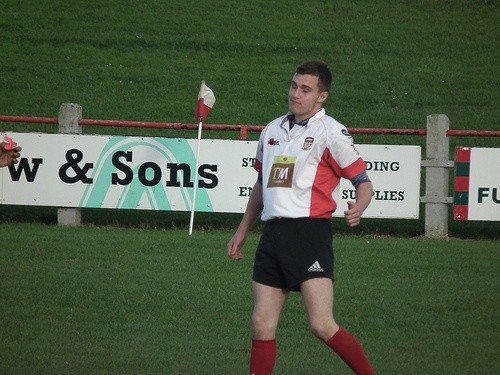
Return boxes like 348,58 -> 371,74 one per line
226,60 -> 378,375
0,140 -> 22,168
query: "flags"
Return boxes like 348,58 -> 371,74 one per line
197,83 -> 216,120
